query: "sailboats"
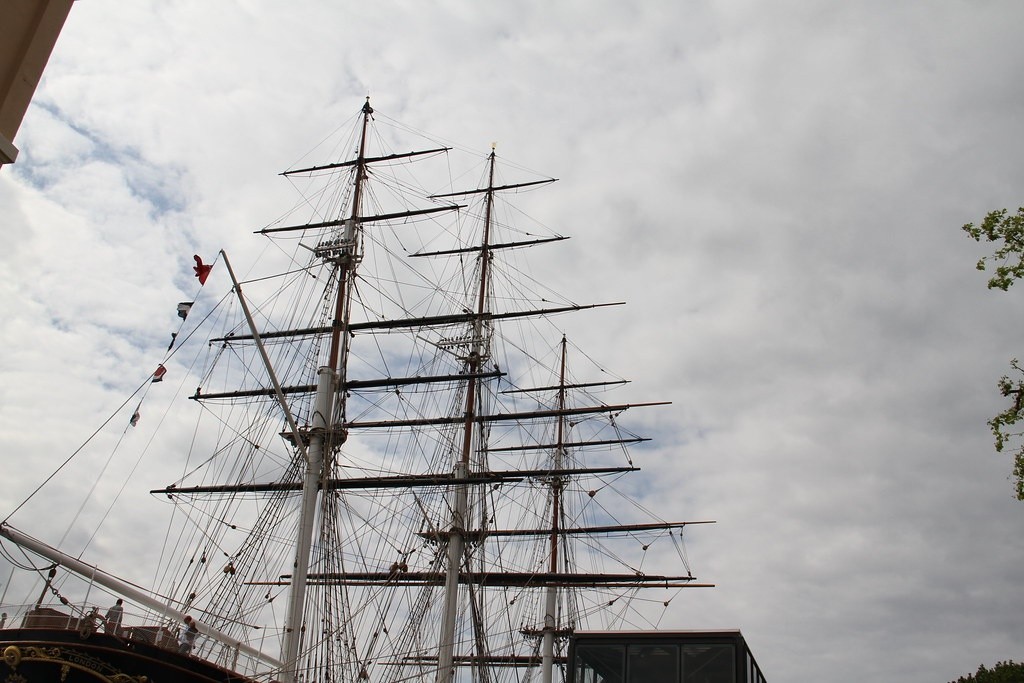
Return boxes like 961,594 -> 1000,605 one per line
0,89 -> 771,683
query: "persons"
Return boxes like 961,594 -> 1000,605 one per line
105,598 -> 123,636
178,622 -> 199,654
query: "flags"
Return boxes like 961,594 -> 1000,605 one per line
176,302 -> 194,322
193,255 -> 213,286
130,409 -> 140,427
152,363 -> 168,383
168,332 -> 177,351
